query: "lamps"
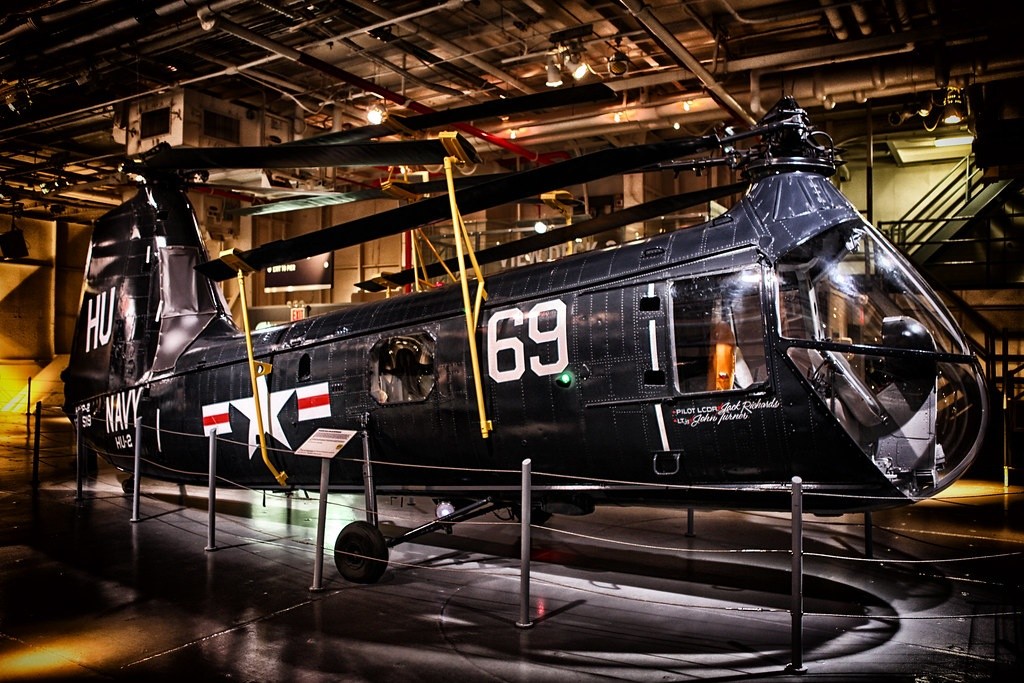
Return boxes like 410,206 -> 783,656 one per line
889,90 -> 964,132
368,100 -> 387,124
545,36 -> 630,88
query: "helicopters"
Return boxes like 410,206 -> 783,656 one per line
60,0 -> 992,585
